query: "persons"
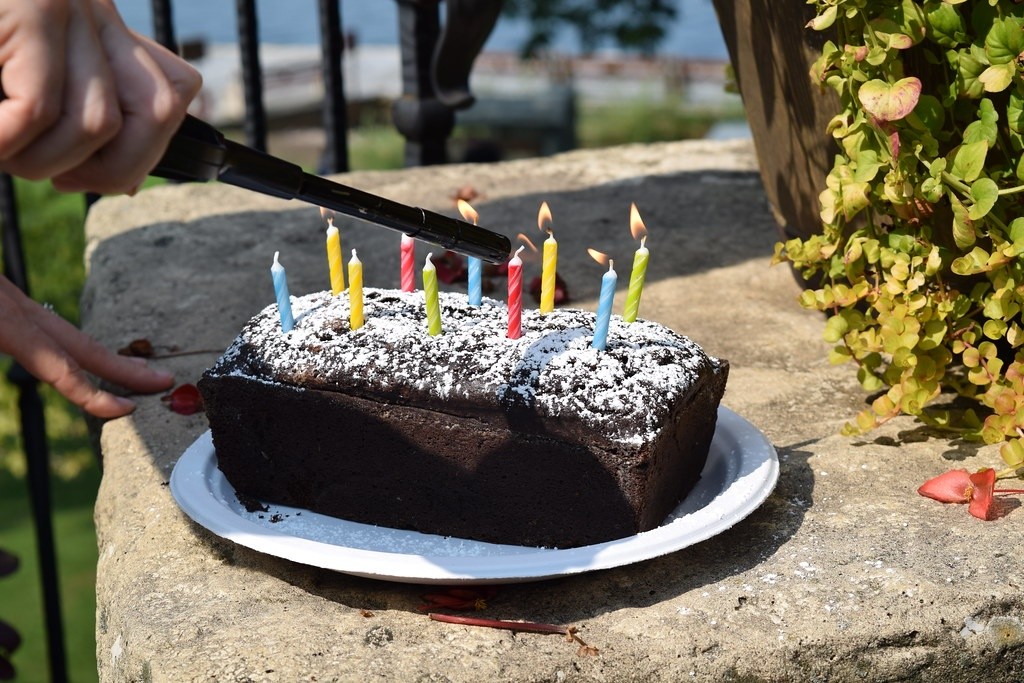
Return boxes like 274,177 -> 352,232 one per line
0,0 -> 202,417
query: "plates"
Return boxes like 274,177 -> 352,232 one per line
167,401 -> 780,586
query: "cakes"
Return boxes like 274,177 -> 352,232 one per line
199,282 -> 730,548
